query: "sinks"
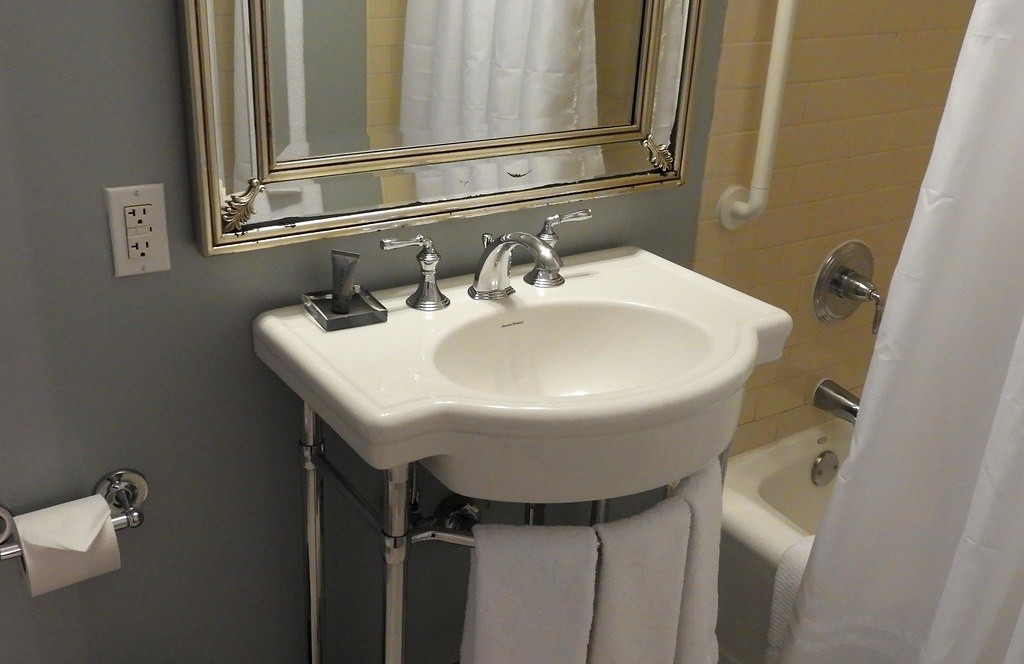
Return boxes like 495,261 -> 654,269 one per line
250,246 -> 794,505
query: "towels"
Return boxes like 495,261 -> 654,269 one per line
590,495 -> 693,664
459,522 -> 600,664
668,454 -> 720,664
761,535 -> 818,664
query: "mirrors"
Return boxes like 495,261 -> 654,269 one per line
174,0 -> 710,258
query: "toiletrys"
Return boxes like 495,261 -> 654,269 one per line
330,248 -> 360,315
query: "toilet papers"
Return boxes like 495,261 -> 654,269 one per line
11,493 -> 122,599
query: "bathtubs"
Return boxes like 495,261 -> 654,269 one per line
715,417 -> 855,664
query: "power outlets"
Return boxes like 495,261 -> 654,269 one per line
104,183 -> 172,278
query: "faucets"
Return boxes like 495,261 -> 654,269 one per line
811,377 -> 862,428
467,232 -> 568,301
